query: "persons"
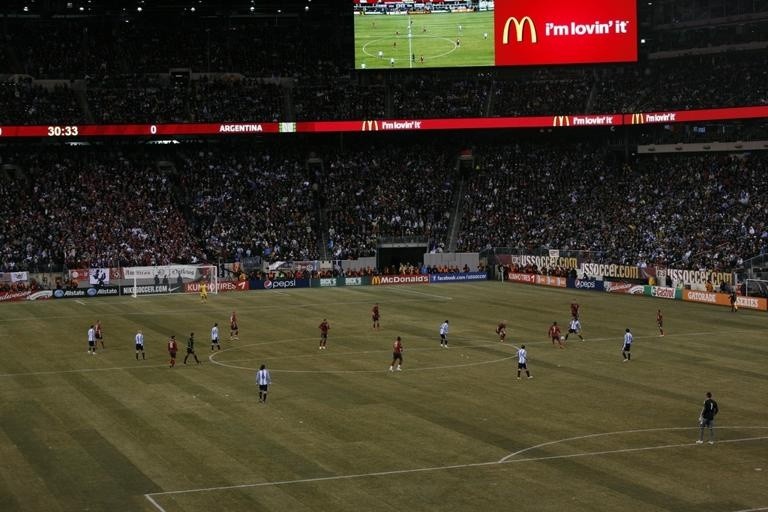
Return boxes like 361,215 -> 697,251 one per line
695,392 -> 719,445
360,21 -> 487,69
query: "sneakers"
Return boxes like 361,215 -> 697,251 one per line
695,439 -> 716,446
516,375 -> 534,380
